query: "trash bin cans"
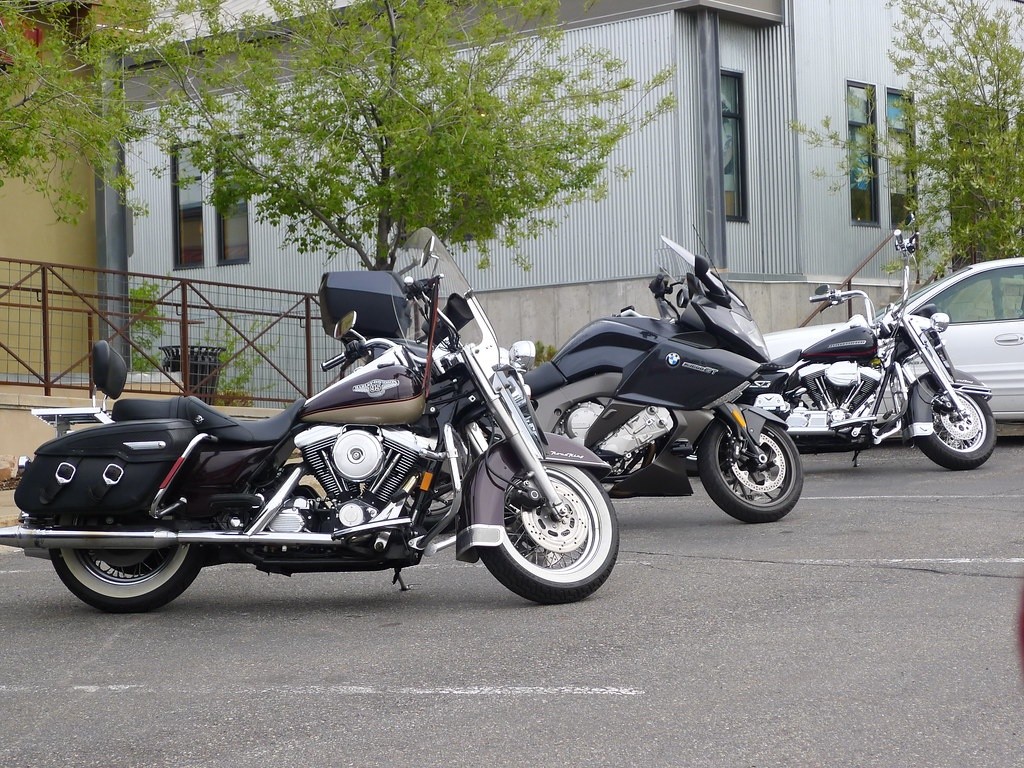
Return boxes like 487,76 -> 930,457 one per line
157,345 -> 228,406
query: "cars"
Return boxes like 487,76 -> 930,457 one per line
762,256 -> 1024,452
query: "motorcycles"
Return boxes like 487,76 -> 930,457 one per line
316,233 -> 806,535
608,210 -> 997,473
1,227 -> 622,604
